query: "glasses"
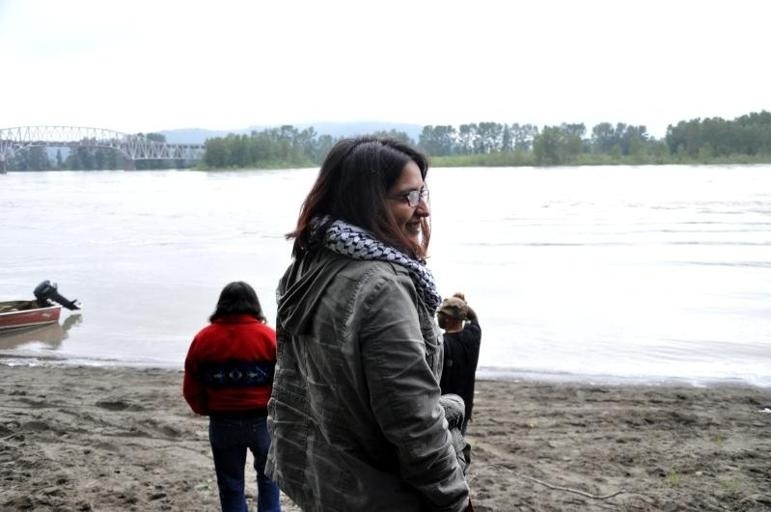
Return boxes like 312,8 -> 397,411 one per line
385,188 -> 429,207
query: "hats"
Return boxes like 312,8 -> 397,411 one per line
439,296 -> 467,319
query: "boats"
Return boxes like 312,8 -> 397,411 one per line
0,279 -> 80,332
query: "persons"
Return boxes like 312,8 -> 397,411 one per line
435,292 -> 484,438
262,134 -> 478,512
178,278 -> 280,510
